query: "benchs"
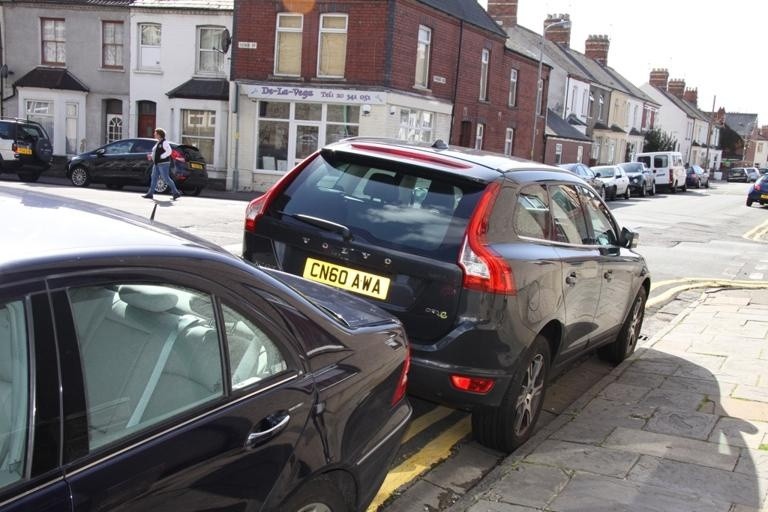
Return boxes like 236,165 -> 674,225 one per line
71,283 -> 260,450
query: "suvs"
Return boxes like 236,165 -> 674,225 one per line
0,114 -> 53,183
243,133 -> 652,458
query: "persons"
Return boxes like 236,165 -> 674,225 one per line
142,128 -> 181,201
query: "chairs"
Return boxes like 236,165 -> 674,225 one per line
0,300 -> 26,488
346,173 -> 397,233
406,181 -> 456,247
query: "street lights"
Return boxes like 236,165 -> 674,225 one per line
530,18 -> 574,158
741,121 -> 762,162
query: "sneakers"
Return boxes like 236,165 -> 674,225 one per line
142,192 -> 153,198
173,192 -> 180,200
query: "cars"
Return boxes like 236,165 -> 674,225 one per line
746,168 -> 760,183
66,137 -> 210,197
297,133 -> 318,148
0,180 -> 412,511
744,173 -> 768,207
616,161 -> 656,198
589,166 -> 633,200
727,167 -> 751,183
757,168 -> 768,175
684,165 -> 709,190
550,162 -> 607,203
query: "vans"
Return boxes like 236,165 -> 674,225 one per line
634,150 -> 688,193
